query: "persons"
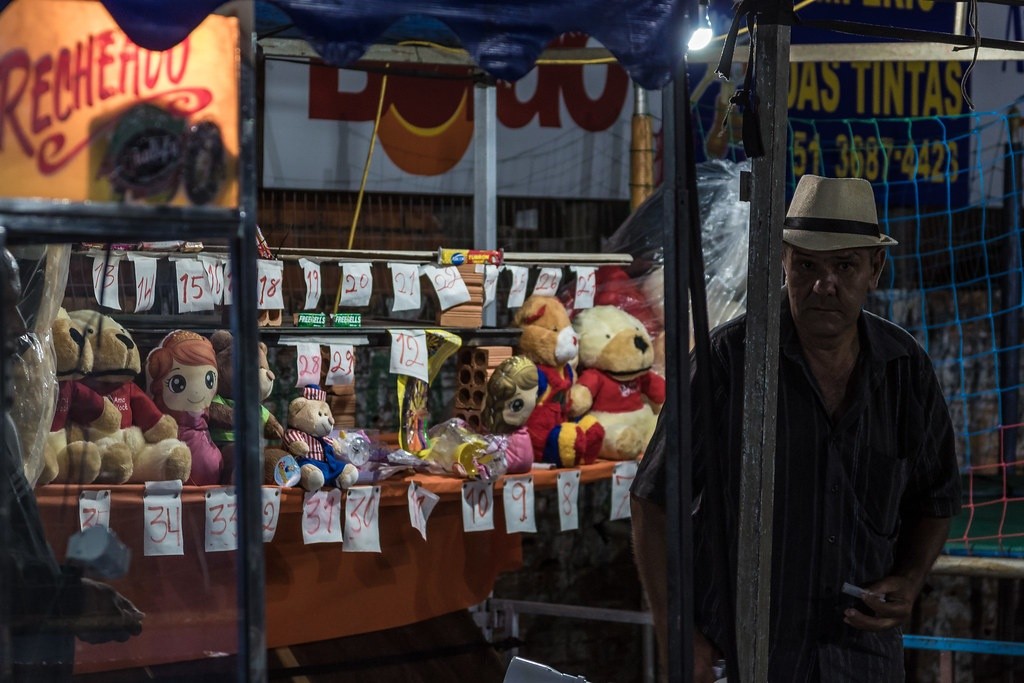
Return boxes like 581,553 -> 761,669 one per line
628,175 -> 963,683
0,225 -> 145,683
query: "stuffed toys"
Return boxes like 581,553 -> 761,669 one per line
145,330 -> 224,486
282,383 -> 359,490
208,330 -> 286,483
69,310 -> 191,483
40,307 -> 134,483
568,305 -> 666,460
482,356 -> 539,475
513,294 -> 605,467
591,163 -> 751,414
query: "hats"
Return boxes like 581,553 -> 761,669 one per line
782,174 -> 899,251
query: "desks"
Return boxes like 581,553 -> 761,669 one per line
486,587 -> 661,683
38,461 -> 638,683
